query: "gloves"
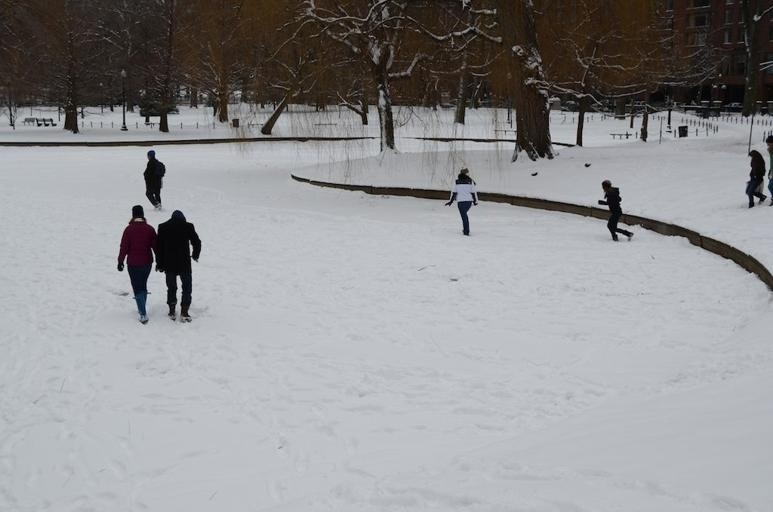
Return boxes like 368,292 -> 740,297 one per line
445,202 -> 452,206
118,263 -> 124,271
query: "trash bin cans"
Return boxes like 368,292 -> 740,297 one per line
678,126 -> 688,137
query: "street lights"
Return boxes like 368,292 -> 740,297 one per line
711,73 -> 726,100
98,82 -> 105,114
118,67 -> 128,132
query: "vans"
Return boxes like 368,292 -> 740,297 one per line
751,100 -> 772,113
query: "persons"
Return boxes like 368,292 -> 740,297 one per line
116,205 -> 160,324
766,135 -> 773,208
598,179 -> 635,241
143,151 -> 166,208
746,150 -> 768,209
157,210 -> 202,322
445,168 -> 478,235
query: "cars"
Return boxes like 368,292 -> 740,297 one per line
724,101 -> 743,109
175,87 -> 244,103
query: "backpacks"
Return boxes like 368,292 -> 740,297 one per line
153,159 -> 165,177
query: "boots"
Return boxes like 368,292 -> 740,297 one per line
139,305 -> 191,323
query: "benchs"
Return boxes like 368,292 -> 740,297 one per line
21,116 -> 34,126
35,118 -> 57,127
